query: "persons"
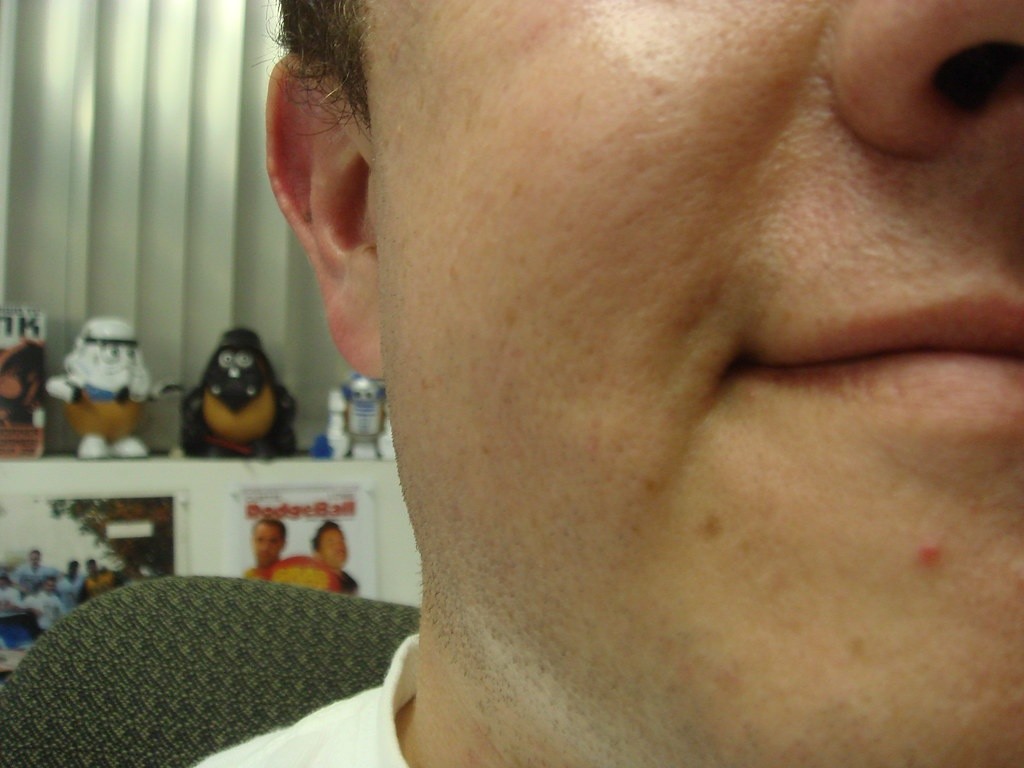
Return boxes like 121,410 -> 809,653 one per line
313,521 -> 358,593
0,0 -> 1024,767
244,518 -> 287,583
0,550 -> 139,652
